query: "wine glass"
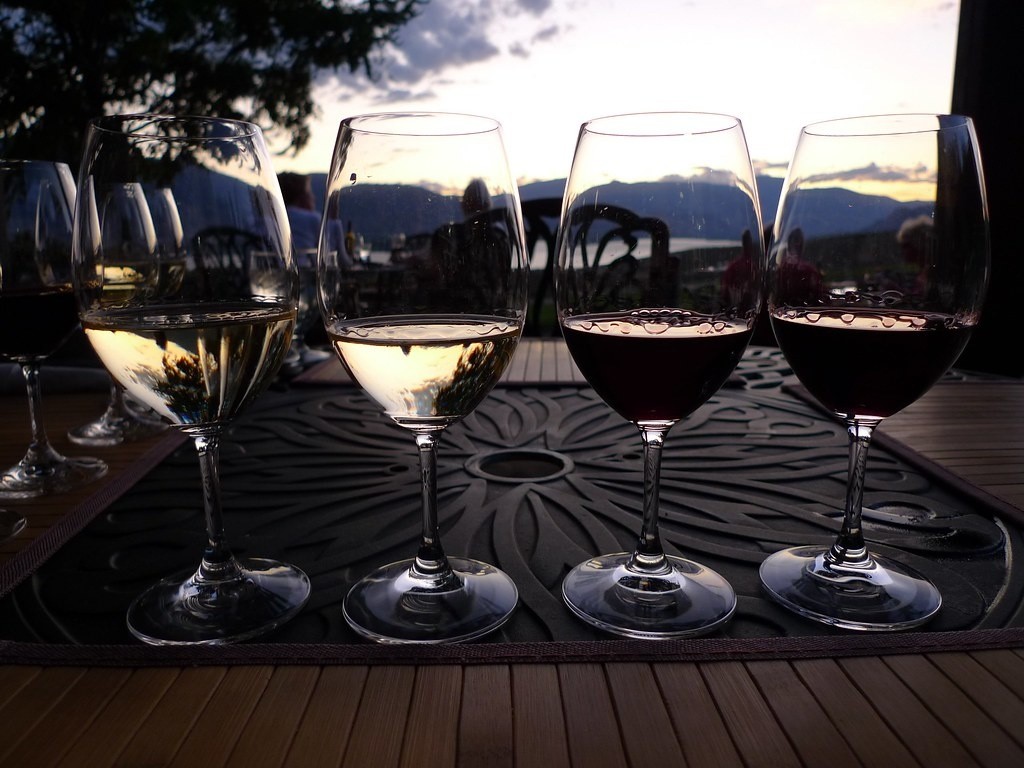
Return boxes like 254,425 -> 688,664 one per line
0,162 -> 163,504
757,116 -> 991,631
314,110 -> 530,645
72,116 -> 312,647
251,245 -> 329,363
554,110 -> 768,640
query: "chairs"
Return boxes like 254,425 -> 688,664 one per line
190,227 -> 286,340
432,196 -> 671,335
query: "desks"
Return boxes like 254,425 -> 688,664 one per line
0,330 -> 1024,768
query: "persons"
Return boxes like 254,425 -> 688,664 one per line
898,215 -> 934,296
722,223 -> 830,306
249,171 -> 511,314
